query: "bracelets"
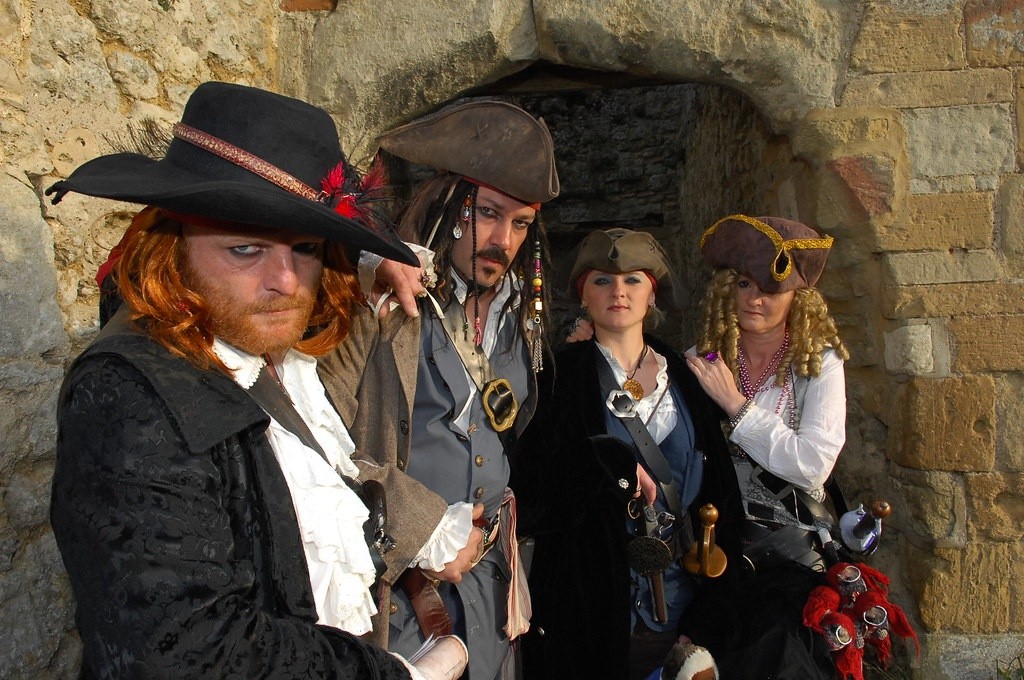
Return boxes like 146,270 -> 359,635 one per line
730,399 -> 756,428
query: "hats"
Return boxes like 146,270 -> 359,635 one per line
374,97 -> 560,211
566,228 -> 678,312
701,214 -> 833,293
45,81 -> 421,268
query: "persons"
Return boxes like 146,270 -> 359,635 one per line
46,81 -> 747,680
549,214 -> 849,680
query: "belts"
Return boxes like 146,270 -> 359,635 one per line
398,513 -> 502,643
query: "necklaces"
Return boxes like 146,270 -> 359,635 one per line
735,325 -> 799,431
624,342 -> 648,401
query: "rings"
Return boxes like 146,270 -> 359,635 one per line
573,317 -> 581,327
704,351 -> 721,364
